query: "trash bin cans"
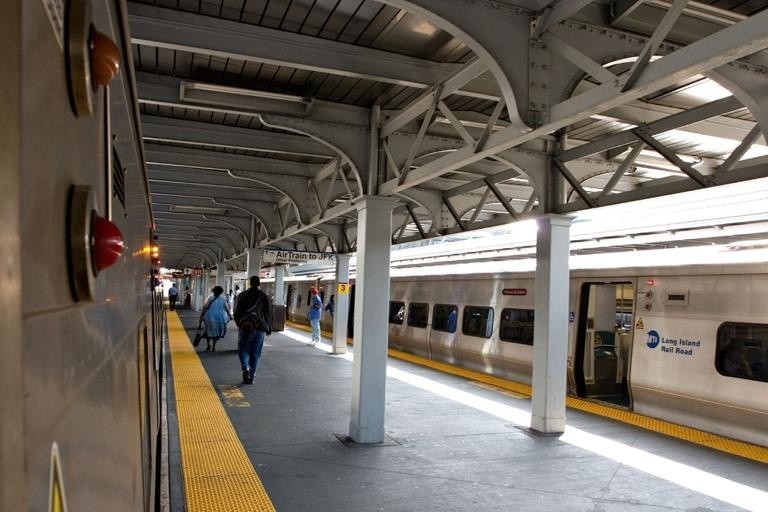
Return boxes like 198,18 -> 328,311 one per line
271,304 -> 287,331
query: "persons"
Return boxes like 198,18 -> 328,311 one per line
199,284 -> 233,353
226,288 -> 234,313
168,282 -> 177,311
182,285 -> 191,308
325,294 -> 334,318
233,275 -> 272,385
306,287 -> 322,346
233,284 -> 242,300
718,336 -> 752,377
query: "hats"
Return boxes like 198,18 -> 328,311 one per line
311,288 -> 319,295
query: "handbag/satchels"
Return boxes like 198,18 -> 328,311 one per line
239,300 -> 269,333
193,334 -> 201,346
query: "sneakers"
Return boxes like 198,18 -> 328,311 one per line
309,341 -> 320,347
243,370 -> 255,384
206,345 -> 217,352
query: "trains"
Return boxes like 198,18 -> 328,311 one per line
1,2 -> 164,511
248,239 -> 767,451
